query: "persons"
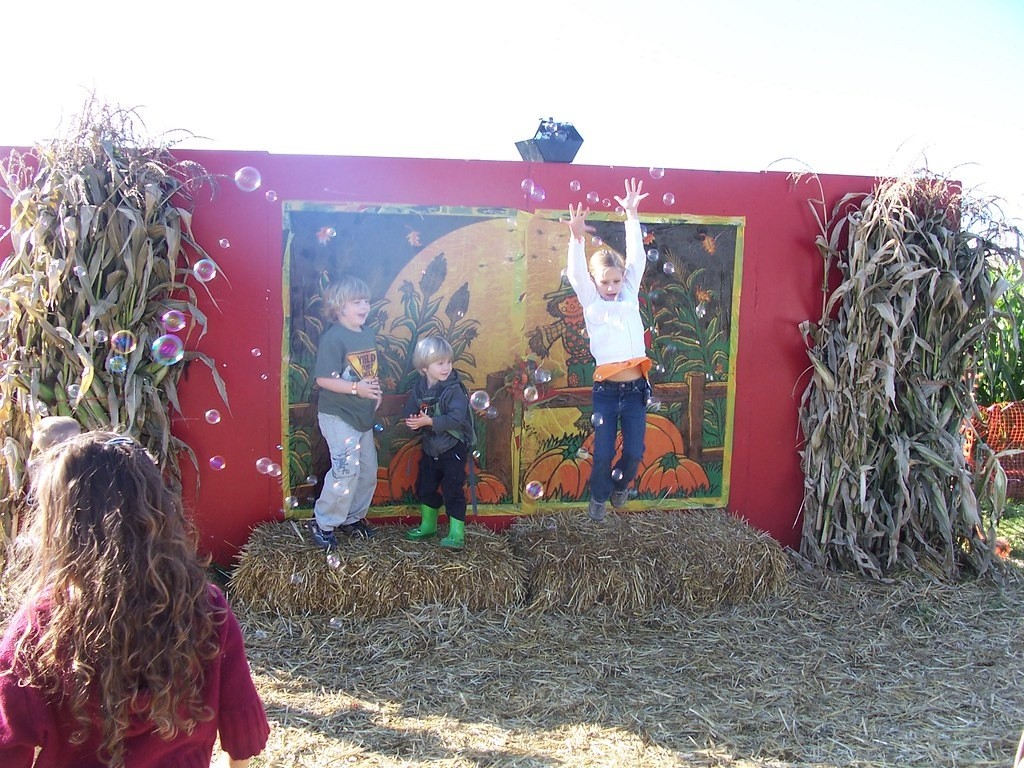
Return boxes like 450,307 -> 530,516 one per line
403,335 -> 473,550
0,416 -> 270,768
308,275 -> 383,548
559,177 -> 652,522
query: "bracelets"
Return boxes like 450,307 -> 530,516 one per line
352,382 -> 357,396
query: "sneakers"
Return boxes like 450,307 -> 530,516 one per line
308,513 -> 340,551
340,520 -> 378,542
588,494 -> 606,521
610,486 -> 629,508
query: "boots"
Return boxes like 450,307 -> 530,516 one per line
405,503 -> 439,540
438,516 -> 465,550
